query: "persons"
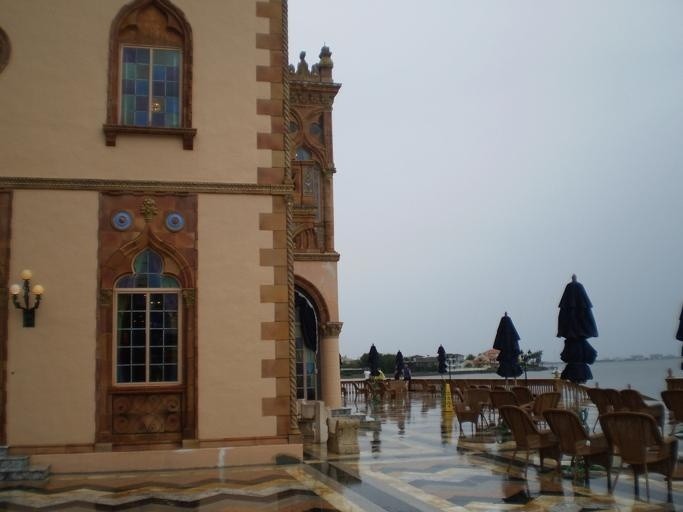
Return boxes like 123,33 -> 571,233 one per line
296,51 -> 311,78
393,366 -> 400,380
375,369 -> 386,400
401,364 -> 411,392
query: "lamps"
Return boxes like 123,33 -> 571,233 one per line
451,384 -> 682,502
352,380 -> 386,403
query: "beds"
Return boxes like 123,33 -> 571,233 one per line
436,344 -> 447,383
366,342 -> 380,382
556,273 -> 600,414
491,310 -> 523,387
393,350 -> 404,370
676,303 -> 683,371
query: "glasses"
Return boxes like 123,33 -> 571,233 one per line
9,269 -> 45,327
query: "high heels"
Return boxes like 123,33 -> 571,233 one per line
441,383 -> 455,412
